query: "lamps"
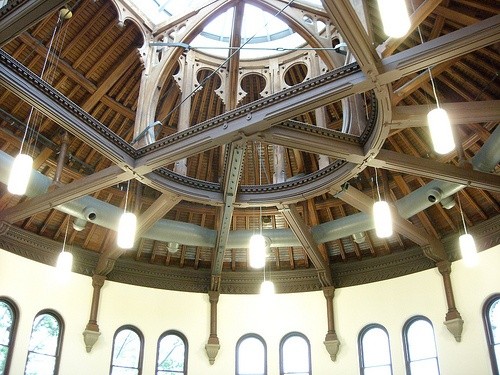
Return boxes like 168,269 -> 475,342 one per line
5,8 -> 74,198
363,92 -> 393,239
55,211 -> 75,281
116,178 -> 138,250
455,191 -> 479,263
256,270 -> 275,308
247,142 -> 267,269
377,0 -> 412,39
410,1 -> 457,155
